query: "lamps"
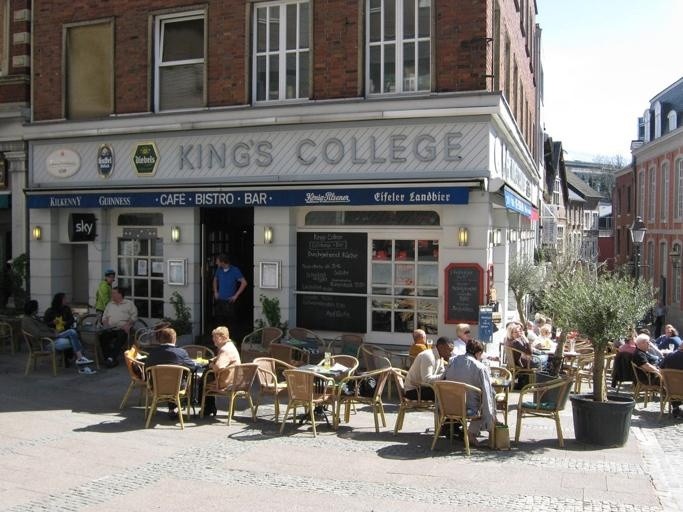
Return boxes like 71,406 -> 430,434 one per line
171,226 -> 180,242
264,226 -> 273,244
458,227 -> 469,246
33,226 -> 41,240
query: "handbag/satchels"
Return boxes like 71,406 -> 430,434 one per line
489,425 -> 510,450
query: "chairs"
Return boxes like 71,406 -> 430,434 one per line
144,365 -> 192,430
278,369 -> 336,437
241,327 -> 283,362
488,367 -> 512,425
317,354 -> 359,422
135,322 -> 172,346
515,376 -> 574,446
22,329 -> 65,376
574,354 -> 616,394
328,334 -> 364,359
270,343 -> 309,382
335,367 -> 392,433
119,349 -> 153,420
0,318 -> 16,355
500,344 -> 543,391
390,368 -> 438,436
429,381 -> 483,454
200,364 -> 261,425
659,369 -> 683,421
401,351 -> 415,369
180,345 -> 215,360
362,344 -> 409,399
612,351 -> 634,393
288,327 -> 325,347
252,358 -> 297,424
631,361 -> 661,408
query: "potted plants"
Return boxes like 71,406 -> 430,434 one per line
534,253 -> 661,447
12,253 -> 28,318
534,327 -> 576,411
164,292 -> 194,345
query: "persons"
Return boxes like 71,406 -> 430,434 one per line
504,322 -> 532,389
451,324 -> 472,357
404,337 -> 462,441
618,335 -> 636,352
20,300 -> 96,374
526,320 -> 538,343
659,343 -> 683,417
409,328 -> 431,357
446,339 -> 496,448
95,269 -> 115,314
145,328 -> 206,421
535,324 -> 556,350
658,329 -> 681,349
656,323 -> 672,343
101,287 -> 138,368
632,334 -> 662,386
533,317 -> 546,335
44,292 -> 75,368
198,326 -> 241,416
213,252 -> 247,326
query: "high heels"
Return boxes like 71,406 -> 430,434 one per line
199,407 -> 217,416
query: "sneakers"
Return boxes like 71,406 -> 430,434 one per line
169,411 -> 180,421
446,433 -> 462,441
78,366 -> 97,375
467,429 -> 482,447
75,356 -> 95,364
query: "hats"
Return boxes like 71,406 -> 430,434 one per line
105,270 -> 115,274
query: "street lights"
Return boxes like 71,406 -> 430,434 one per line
627,215 -> 646,282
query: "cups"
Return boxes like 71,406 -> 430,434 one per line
324,352 -> 331,369
427,339 -> 433,350
669,343 -> 675,352
197,350 -> 202,363
494,369 -> 500,380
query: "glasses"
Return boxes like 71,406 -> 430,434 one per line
545,331 -> 551,334
512,331 -> 522,333
642,341 -> 650,345
464,330 -> 470,334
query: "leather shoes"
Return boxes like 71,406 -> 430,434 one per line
103,358 -> 119,368
673,407 -> 680,417
